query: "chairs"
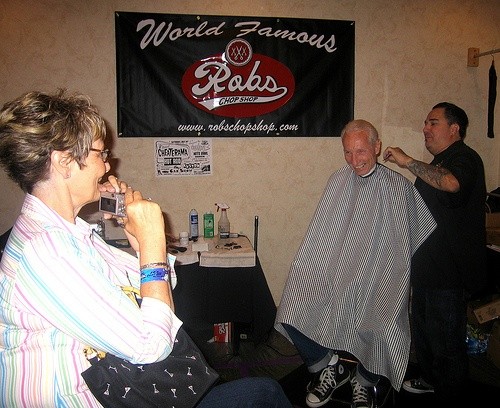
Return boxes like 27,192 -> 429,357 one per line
307,284 -> 413,408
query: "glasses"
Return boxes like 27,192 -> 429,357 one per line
76,145 -> 111,162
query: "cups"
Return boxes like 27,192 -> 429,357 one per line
179,232 -> 188,246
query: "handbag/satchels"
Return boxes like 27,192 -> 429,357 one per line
80,287 -> 220,408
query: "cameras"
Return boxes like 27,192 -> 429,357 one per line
98,191 -> 127,217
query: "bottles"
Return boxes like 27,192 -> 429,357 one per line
189,208 -> 198,238
204,211 -> 214,238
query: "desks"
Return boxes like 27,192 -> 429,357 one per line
173,235 -> 304,388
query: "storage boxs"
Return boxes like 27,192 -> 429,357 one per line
199,234 -> 255,268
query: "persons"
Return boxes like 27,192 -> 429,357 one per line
272,120 -> 437,408
0,87 -> 293,408
382,102 -> 487,408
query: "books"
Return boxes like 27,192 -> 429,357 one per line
166,236 -> 256,267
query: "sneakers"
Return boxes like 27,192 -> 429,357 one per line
306,357 -> 349,408
404,378 -> 435,393
350,372 -> 375,408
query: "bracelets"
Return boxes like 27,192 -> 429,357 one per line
139,261 -> 169,270
139,267 -> 170,284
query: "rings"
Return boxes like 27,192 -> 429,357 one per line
145,198 -> 153,201
